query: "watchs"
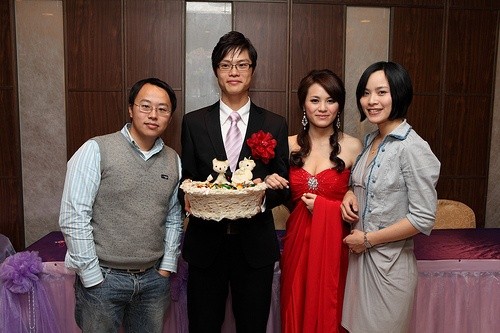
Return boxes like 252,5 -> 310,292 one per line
363,231 -> 372,248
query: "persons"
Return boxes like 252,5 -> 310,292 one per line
177,30 -> 289,333
58,78 -> 182,333
339,60 -> 441,333
264,69 -> 363,333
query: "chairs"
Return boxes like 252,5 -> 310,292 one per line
435,199 -> 476,228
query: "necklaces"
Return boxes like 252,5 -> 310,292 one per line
371,148 -> 376,154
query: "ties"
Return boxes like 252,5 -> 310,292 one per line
225,112 -> 242,173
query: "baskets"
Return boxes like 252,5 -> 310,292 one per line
180,180 -> 267,221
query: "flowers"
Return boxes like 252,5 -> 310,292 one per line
245,130 -> 278,164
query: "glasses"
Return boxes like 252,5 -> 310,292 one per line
217,61 -> 253,71
133,102 -> 172,115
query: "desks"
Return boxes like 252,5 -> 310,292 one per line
0,228 -> 500,333
